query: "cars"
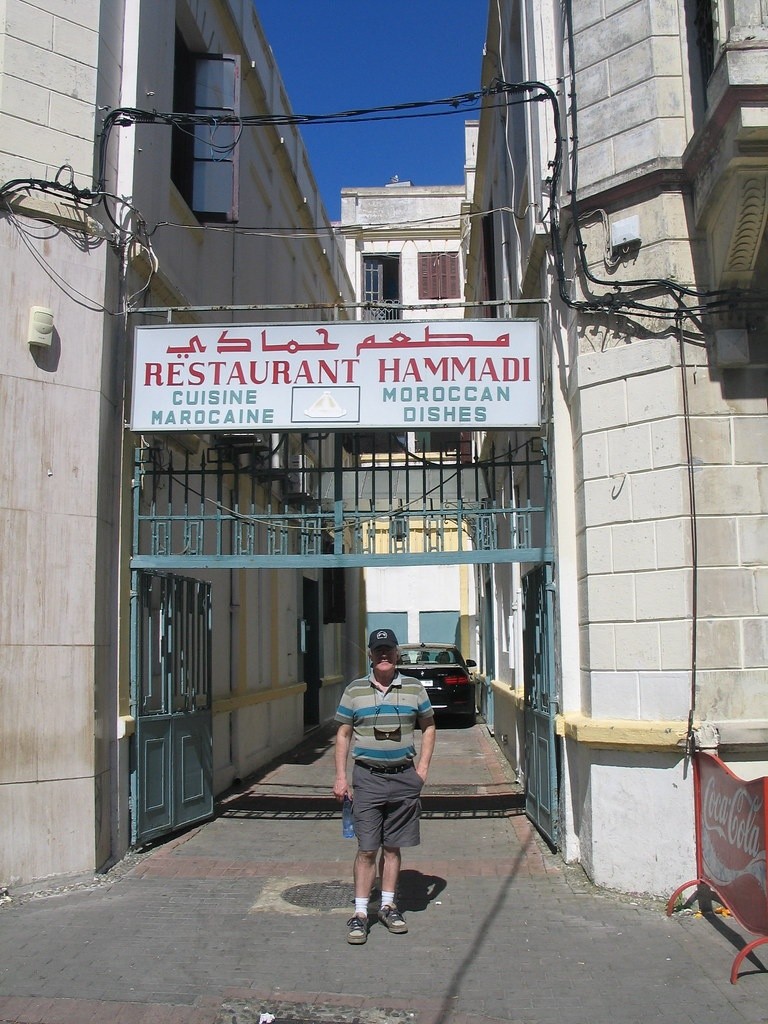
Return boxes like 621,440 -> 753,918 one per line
370,643 -> 477,725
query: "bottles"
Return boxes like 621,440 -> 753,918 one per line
342,795 -> 355,837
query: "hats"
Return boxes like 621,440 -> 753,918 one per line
368,629 -> 398,650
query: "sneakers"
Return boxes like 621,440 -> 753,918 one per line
377,903 -> 408,933
346,912 -> 369,943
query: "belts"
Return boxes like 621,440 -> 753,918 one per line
355,759 -> 414,774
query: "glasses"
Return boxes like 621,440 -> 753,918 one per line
373,707 -> 401,734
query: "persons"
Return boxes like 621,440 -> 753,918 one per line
333,629 -> 435,944
